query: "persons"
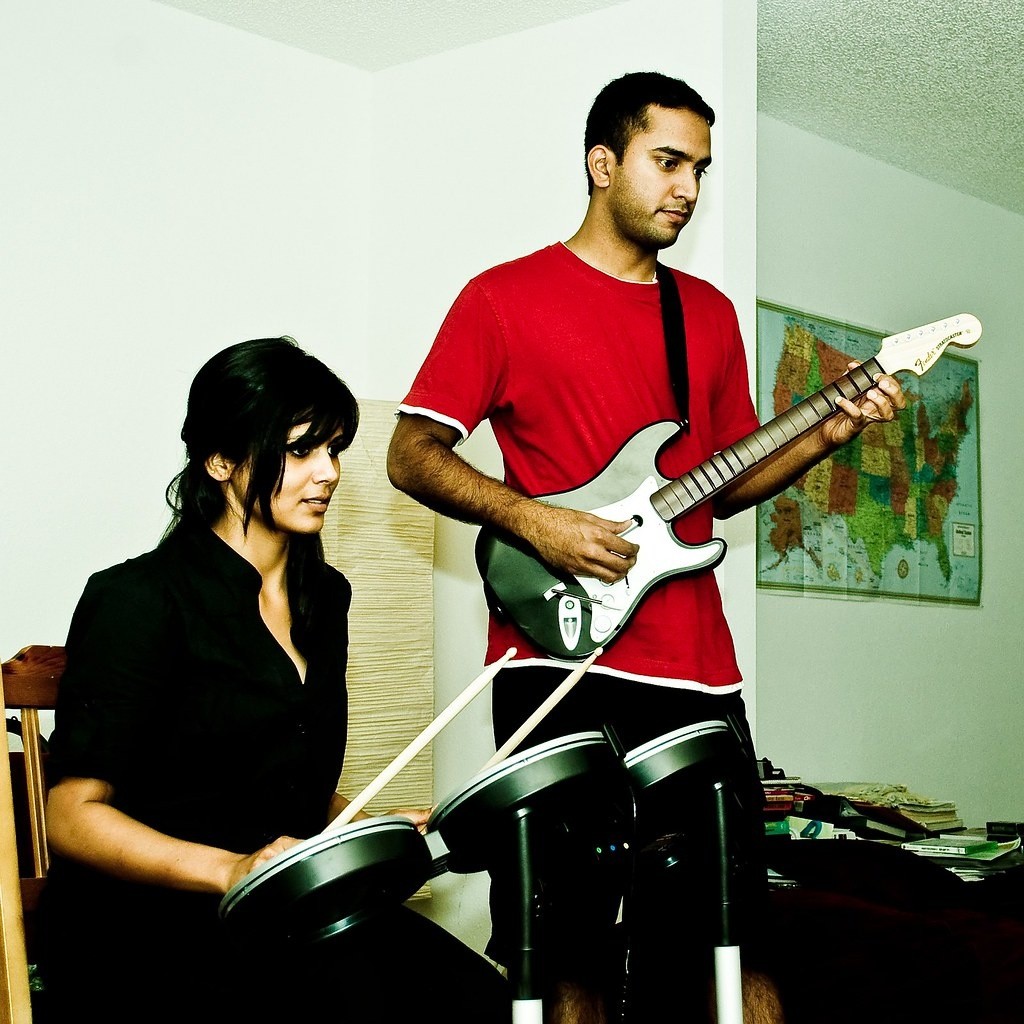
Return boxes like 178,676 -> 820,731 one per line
36,336 -> 514,1023
388,70 -> 909,1024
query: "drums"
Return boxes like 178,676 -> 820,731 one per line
216,812 -> 434,950
427,720 -> 629,874
624,720 -> 751,823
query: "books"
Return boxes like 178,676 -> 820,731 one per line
758,775 -> 1022,883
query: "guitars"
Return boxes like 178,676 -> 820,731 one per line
473,312 -> 983,662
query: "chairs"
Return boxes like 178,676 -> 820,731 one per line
0,647 -> 88,1024
